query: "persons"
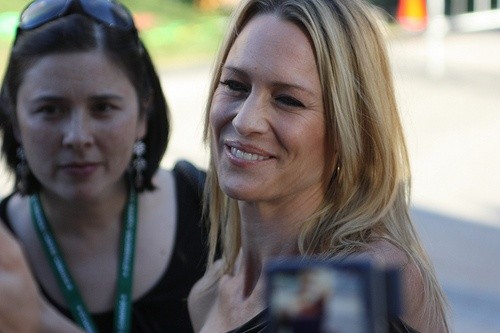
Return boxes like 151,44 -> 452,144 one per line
0,0 -> 454,333
0,1 -> 222,333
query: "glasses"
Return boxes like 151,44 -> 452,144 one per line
12,0 -> 141,51
